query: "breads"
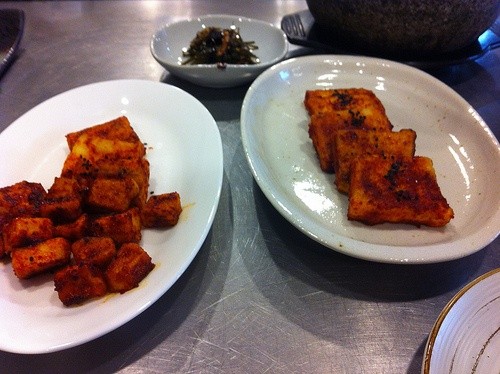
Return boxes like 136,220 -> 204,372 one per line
305,86 -> 454,226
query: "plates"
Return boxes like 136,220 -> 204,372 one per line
281,9 -> 499,54
150,16 -> 288,88
0,9 -> 25,74
0,80 -> 224,353
240,55 -> 499,264
422,264 -> 499,374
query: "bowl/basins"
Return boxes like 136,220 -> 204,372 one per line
305,0 -> 500,46
